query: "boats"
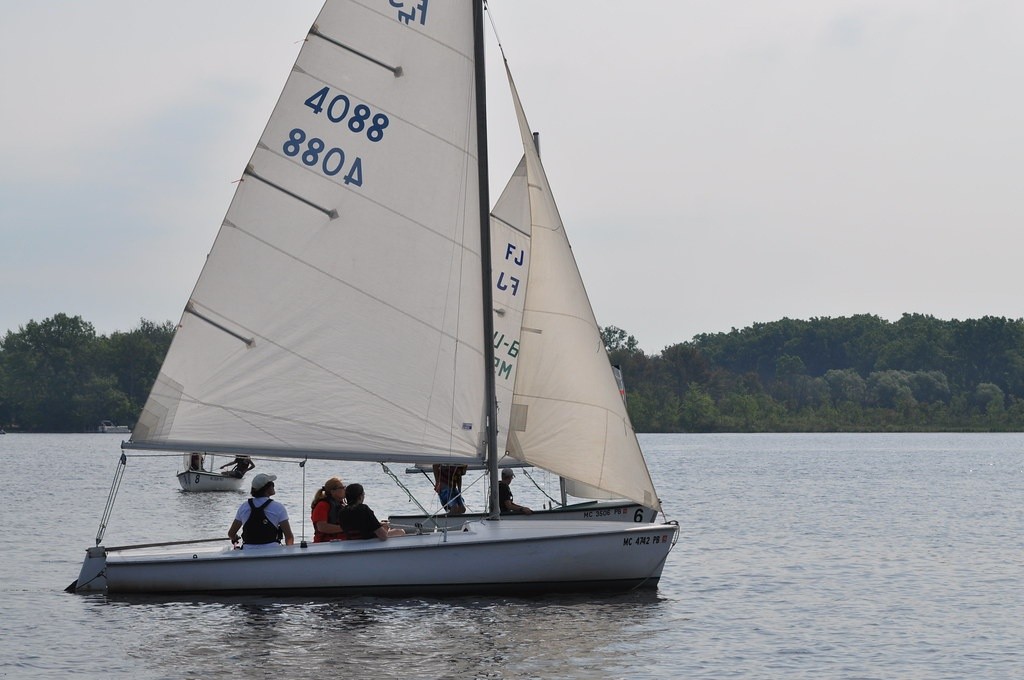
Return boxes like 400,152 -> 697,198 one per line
100,421 -> 131,434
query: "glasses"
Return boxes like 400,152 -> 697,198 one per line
333,486 -> 347,491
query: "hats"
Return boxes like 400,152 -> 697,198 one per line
502,468 -> 516,477
251,474 -> 277,491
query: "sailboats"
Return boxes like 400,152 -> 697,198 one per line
64,0 -> 682,589
177,454 -> 246,492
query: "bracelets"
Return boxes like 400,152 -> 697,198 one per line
520,507 -> 524,511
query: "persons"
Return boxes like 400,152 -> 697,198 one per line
228,474 -> 294,546
433,464 -> 467,515
338,483 -> 404,540
220,455 -> 255,478
499,468 -> 533,514
191,453 -> 204,470
310,476 -> 347,541
